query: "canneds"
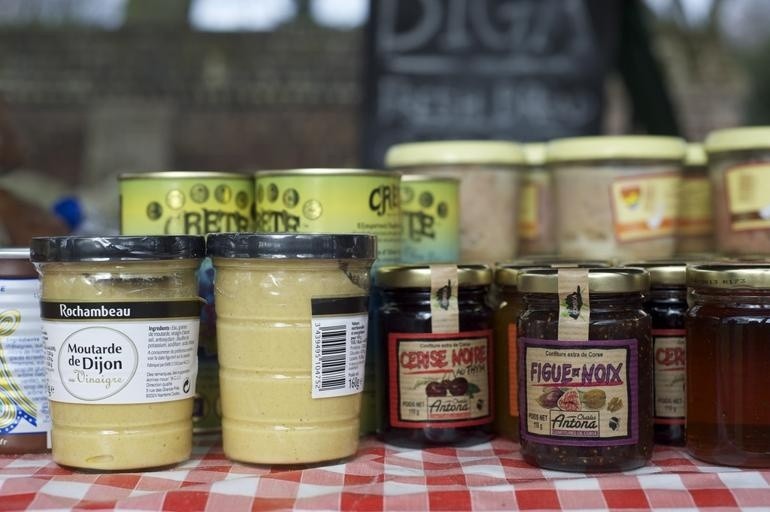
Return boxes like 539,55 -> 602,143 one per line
0,125 -> 770,472
